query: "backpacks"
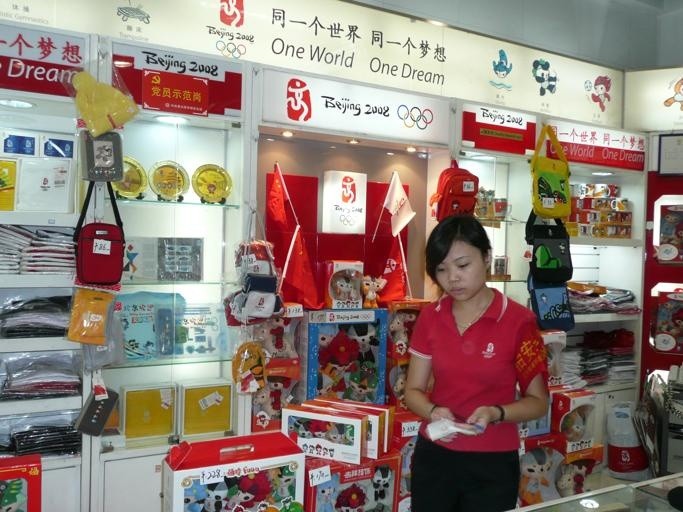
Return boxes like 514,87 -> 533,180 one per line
431,160 -> 478,222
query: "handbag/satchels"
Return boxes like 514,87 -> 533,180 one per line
76,224 -> 123,284
530,156 -> 575,331
224,208 -> 285,394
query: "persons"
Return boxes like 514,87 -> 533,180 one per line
401,214 -> 548,512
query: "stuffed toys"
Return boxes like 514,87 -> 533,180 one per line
516,394 -> 596,509
183,272 -> 417,512
657,212 -> 683,342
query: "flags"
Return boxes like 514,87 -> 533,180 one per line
383,173 -> 417,236
263,164 -> 298,266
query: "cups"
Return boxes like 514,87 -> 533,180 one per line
567,180 -> 631,238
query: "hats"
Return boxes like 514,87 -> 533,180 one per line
71,72 -> 137,137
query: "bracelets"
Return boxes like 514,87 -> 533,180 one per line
497,405 -> 505,424
428,404 -> 438,422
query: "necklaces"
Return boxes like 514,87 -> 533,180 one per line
452,288 -> 492,329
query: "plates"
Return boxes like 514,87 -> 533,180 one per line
110,155 -> 144,196
191,164 -> 231,202
147,158 -> 190,201
